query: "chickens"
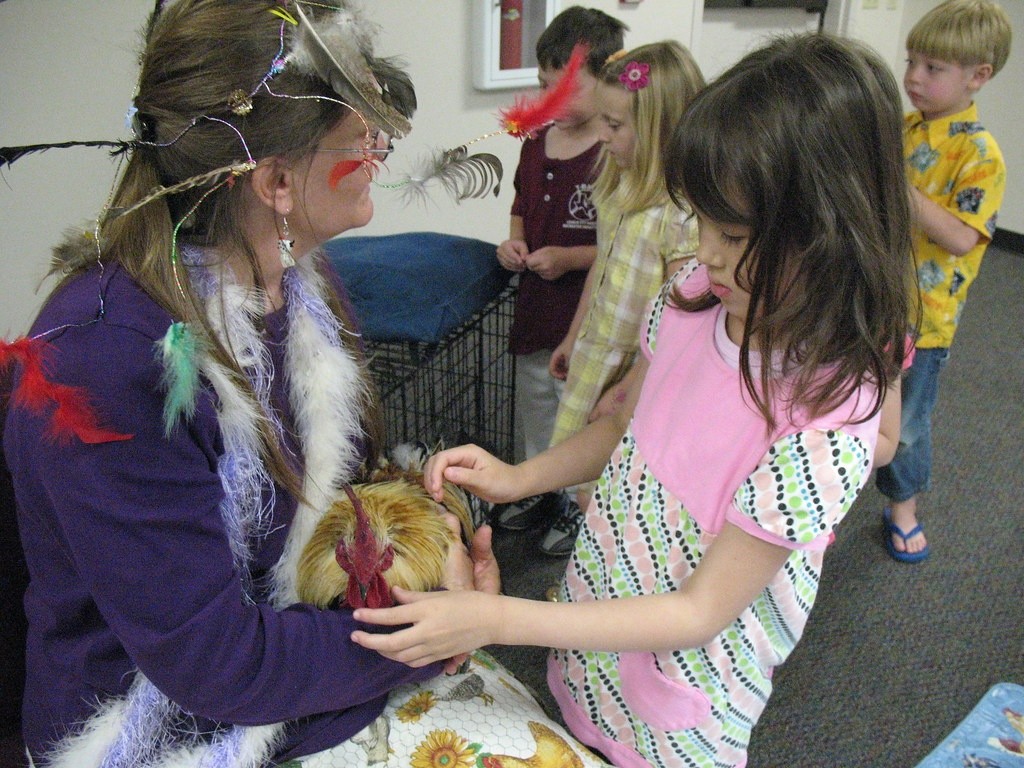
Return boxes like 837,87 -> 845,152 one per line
295,449 -> 475,611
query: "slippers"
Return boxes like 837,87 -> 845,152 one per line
884,506 -> 929,561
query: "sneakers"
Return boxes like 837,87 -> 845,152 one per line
539,501 -> 584,556
495,487 -> 561,531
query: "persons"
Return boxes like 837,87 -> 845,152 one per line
495,7 -> 631,556
548,41 -> 706,516
350,34 -> 921,768
876,0 -> 1012,563
0,0 -> 502,768
